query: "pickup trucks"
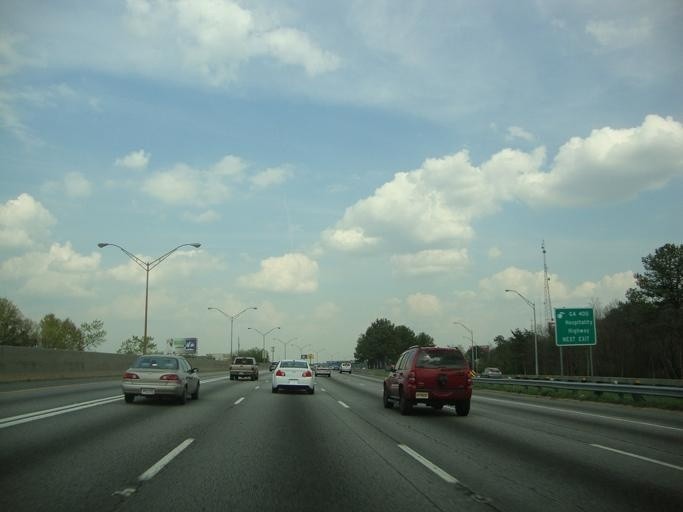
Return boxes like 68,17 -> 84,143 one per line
229,356 -> 260,381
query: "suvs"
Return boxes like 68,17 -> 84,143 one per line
382,343 -> 476,417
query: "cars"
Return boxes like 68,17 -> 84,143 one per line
269,361 -> 280,371
271,358 -> 315,395
315,365 -> 330,377
119,354 -> 201,405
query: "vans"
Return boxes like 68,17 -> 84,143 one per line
339,362 -> 352,374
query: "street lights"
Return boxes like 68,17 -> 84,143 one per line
248,327 -> 279,361
248,326 -> 281,364
272,336 -> 296,360
292,342 -> 312,360
